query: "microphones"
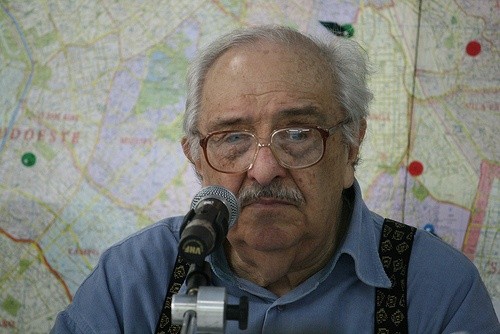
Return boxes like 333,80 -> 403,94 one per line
179,185 -> 240,263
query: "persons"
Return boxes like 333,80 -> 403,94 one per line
50,23 -> 500,334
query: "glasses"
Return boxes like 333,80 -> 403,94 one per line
191,118 -> 351,174
